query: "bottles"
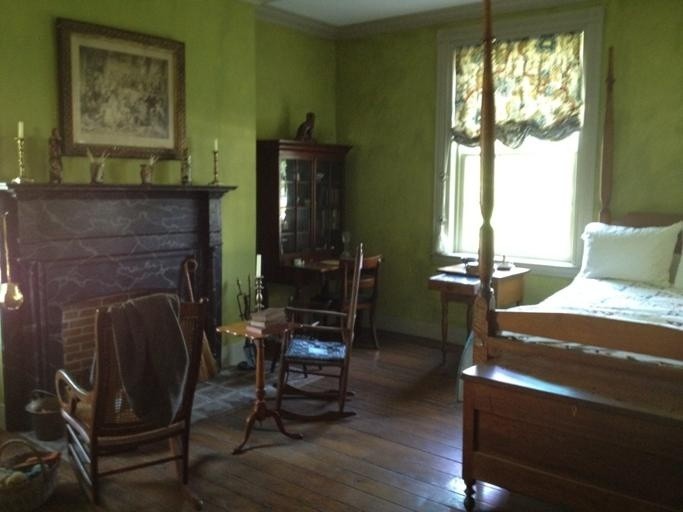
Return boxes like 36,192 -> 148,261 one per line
179,136 -> 192,185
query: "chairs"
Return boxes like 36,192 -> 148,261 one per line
51,293 -> 210,505
276,242 -> 364,421
308,250 -> 386,352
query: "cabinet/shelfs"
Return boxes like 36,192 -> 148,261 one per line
259,142 -> 348,284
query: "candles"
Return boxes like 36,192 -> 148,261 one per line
256,252 -> 261,277
17,121 -> 25,135
212,138 -> 217,150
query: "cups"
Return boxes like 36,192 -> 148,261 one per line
140,163 -> 153,184
294,257 -> 304,265
90,161 -> 105,184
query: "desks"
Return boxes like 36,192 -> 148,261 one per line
215,319 -> 304,455
429,263 -> 530,367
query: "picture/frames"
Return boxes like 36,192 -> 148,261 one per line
51,18 -> 190,160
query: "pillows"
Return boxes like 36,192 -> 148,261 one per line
581,215 -> 683,288
674,257 -> 681,294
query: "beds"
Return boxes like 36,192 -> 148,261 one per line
459,0 -> 682,511
